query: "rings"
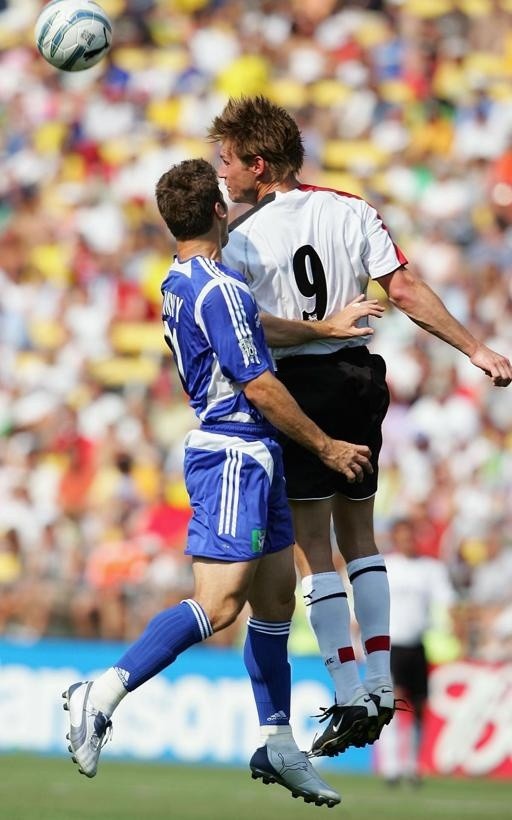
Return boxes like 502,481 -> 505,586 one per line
351,302 -> 362,308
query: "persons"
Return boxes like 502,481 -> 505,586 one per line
0,0 -> 510,668
350,516 -> 461,792
60,157 -> 388,806
208,93 -> 511,757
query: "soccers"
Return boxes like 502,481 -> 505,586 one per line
35,0 -> 112,71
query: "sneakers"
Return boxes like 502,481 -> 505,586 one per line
310,685 -> 397,759
245,729 -> 341,808
62,679 -> 112,779
